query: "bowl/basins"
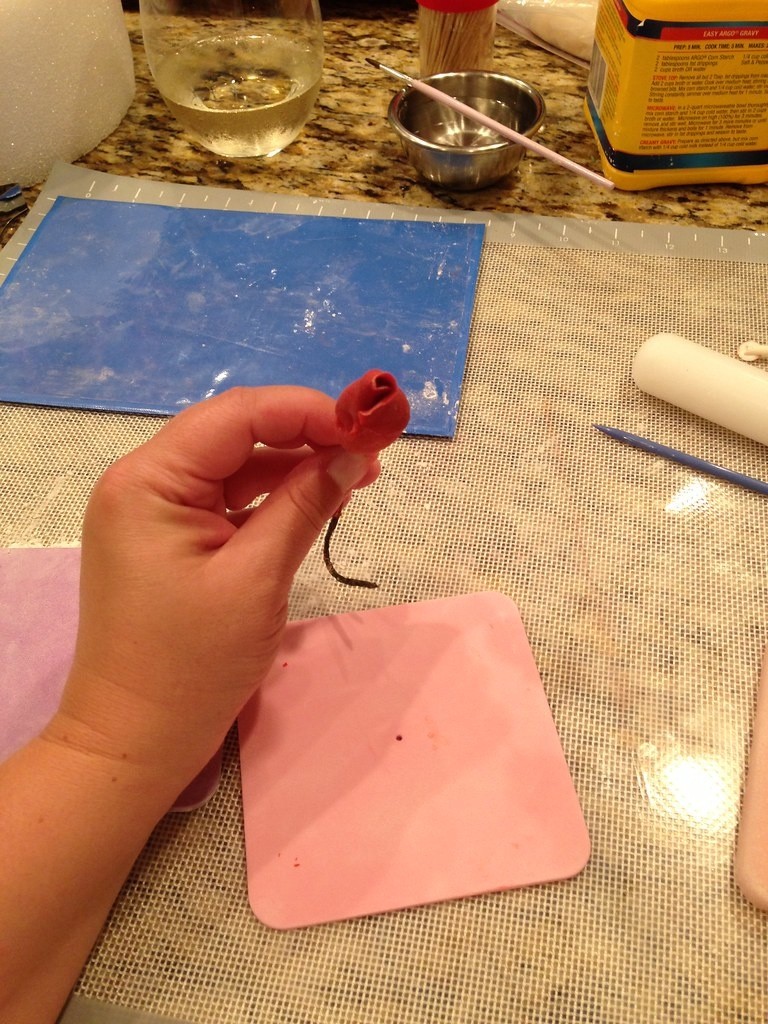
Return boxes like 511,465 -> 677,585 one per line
388,69 -> 546,191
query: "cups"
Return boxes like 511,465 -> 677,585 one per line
416,0 -> 499,78
138,0 -> 323,160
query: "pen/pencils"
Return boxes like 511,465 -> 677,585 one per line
589,418 -> 768,514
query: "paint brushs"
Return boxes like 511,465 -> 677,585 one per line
364,53 -> 616,197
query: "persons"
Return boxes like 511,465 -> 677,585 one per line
1,385 -> 382,1024
